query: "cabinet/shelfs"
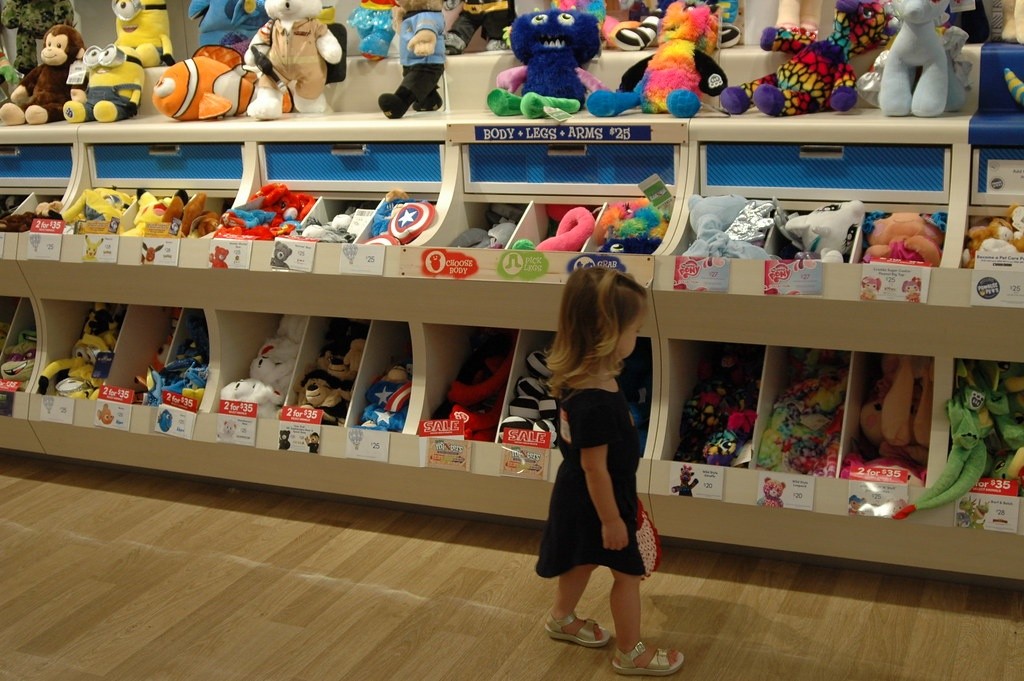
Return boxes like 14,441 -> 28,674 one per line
1,40 -> 1023,586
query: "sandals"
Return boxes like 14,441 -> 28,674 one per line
612,639 -> 683,675
544,610 -> 610,648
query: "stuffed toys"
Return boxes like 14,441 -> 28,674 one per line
0,24 -> 85,125
347,0 -> 519,119
0,187 -> 1024,269
0,300 -> 1024,519
111,0 -> 175,68
63,45 -> 145,123
486,0 -> 1024,119
151,1 -> 347,121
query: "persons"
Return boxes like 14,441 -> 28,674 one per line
537,267 -> 683,674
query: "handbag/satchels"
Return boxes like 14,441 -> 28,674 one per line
636,499 -> 661,582
